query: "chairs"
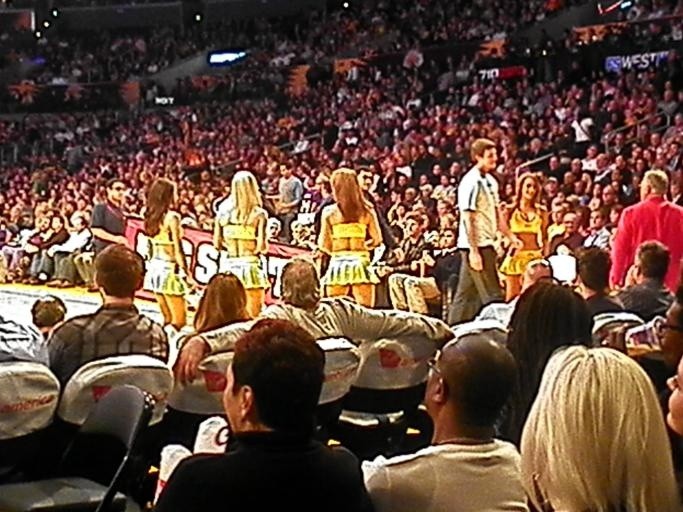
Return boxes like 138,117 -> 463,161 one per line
2,384 -> 147,512
1,313 -> 666,459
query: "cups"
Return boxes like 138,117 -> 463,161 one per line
189,414 -> 228,459
621,317 -> 665,353
154,444 -> 192,506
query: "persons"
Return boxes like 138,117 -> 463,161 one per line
212,170 -> 270,318
520,344 -> 682,511
476,260 -> 553,327
168,272 -> 254,368
2,315 -> 50,367
363,333 -> 529,512
31,295 -> 68,340
609,170 -> 683,294
574,245 -> 625,317
616,240 -> 677,323
88,179 -> 129,254
609,286 -> 683,365
318,168 -> 382,306
47,243 -> 169,382
144,178 -> 195,337
667,358 -> 682,434
154,319 -> 376,512
447,138 -> 523,327
490,282 -> 593,454
175,258 -> 456,385
0,0 -> 683,293
